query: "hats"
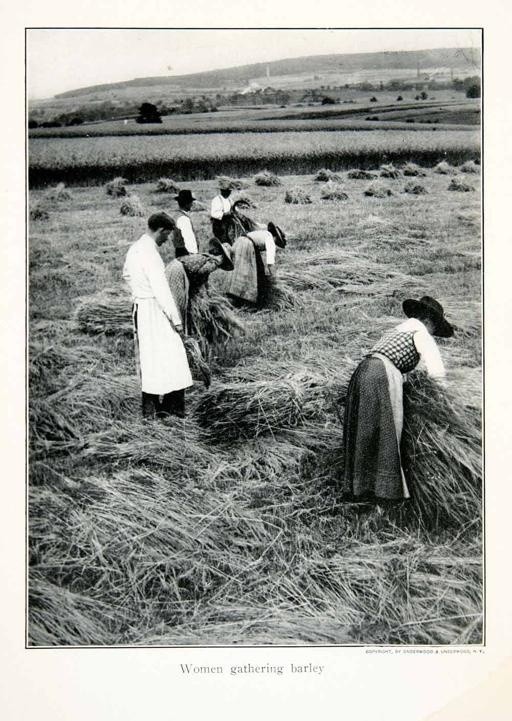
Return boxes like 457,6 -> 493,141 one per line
403,296 -> 453,337
175,190 -> 197,201
268,222 -> 285,249
210,238 -> 234,271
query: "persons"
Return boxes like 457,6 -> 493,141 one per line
344,297 -> 453,510
122,212 -> 194,419
209,182 -> 243,243
165,237 -> 237,331
171,189 -> 199,256
228,221 -> 286,305
